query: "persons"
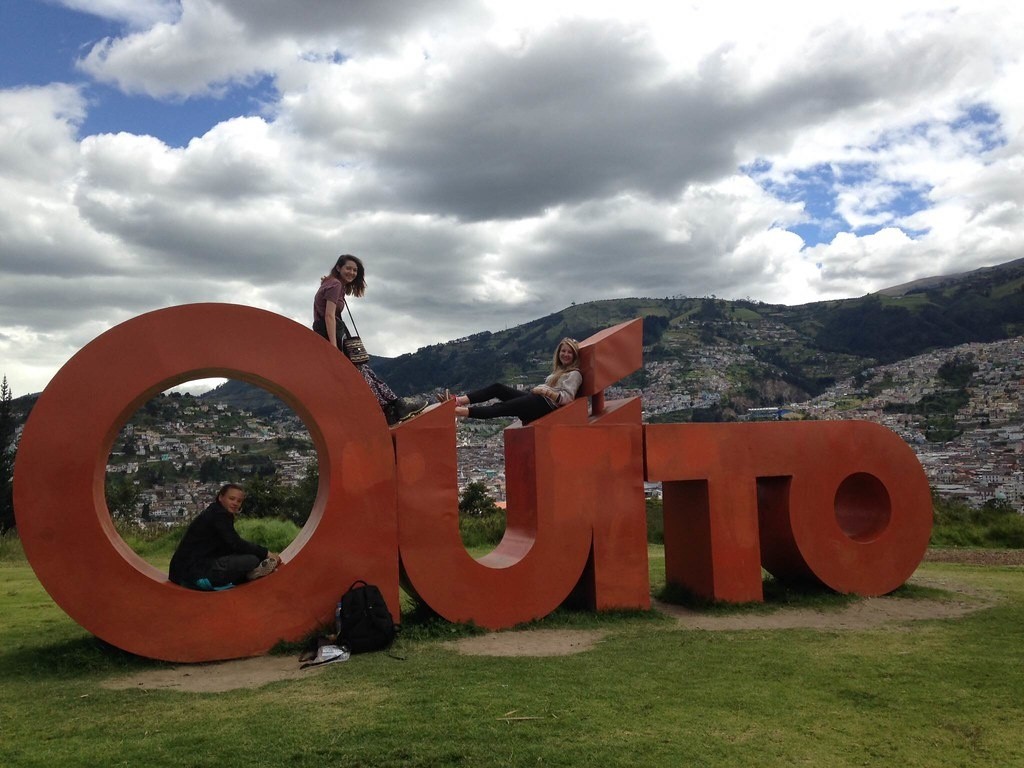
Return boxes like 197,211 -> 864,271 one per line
169,485 -> 281,590
313,254 -> 428,425
436,338 -> 582,422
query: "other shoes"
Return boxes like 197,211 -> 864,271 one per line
247,557 -> 277,580
393,397 -> 429,421
436,393 -> 448,403
384,405 -> 402,427
445,389 -> 453,401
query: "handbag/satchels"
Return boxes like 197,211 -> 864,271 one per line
344,336 -> 369,364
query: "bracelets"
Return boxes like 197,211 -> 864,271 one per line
545,389 -> 553,397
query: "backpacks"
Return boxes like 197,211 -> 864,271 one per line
298,580 -> 411,669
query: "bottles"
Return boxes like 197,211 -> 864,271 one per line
335,602 -> 343,634
